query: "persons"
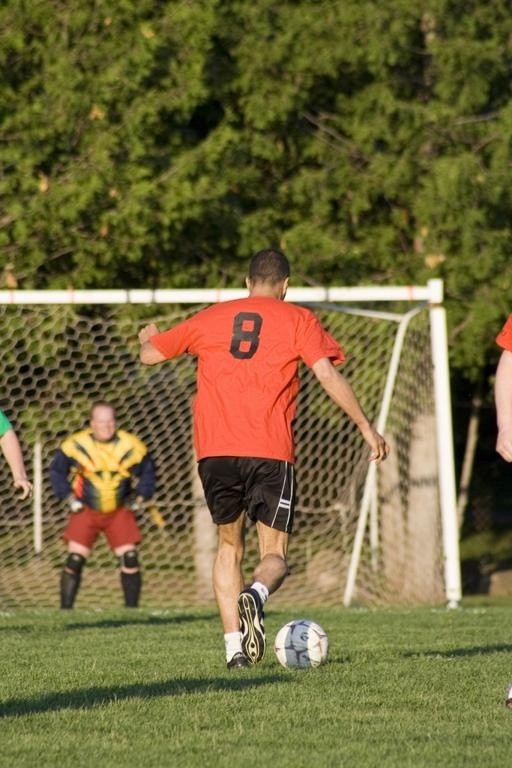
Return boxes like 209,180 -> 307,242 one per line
48,400 -> 157,608
491,311 -> 512,462
136,247 -> 392,667
1,411 -> 34,503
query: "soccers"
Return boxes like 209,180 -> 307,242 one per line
273,619 -> 328,668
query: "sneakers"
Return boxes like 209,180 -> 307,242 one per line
235,589 -> 266,666
228,653 -> 249,673
